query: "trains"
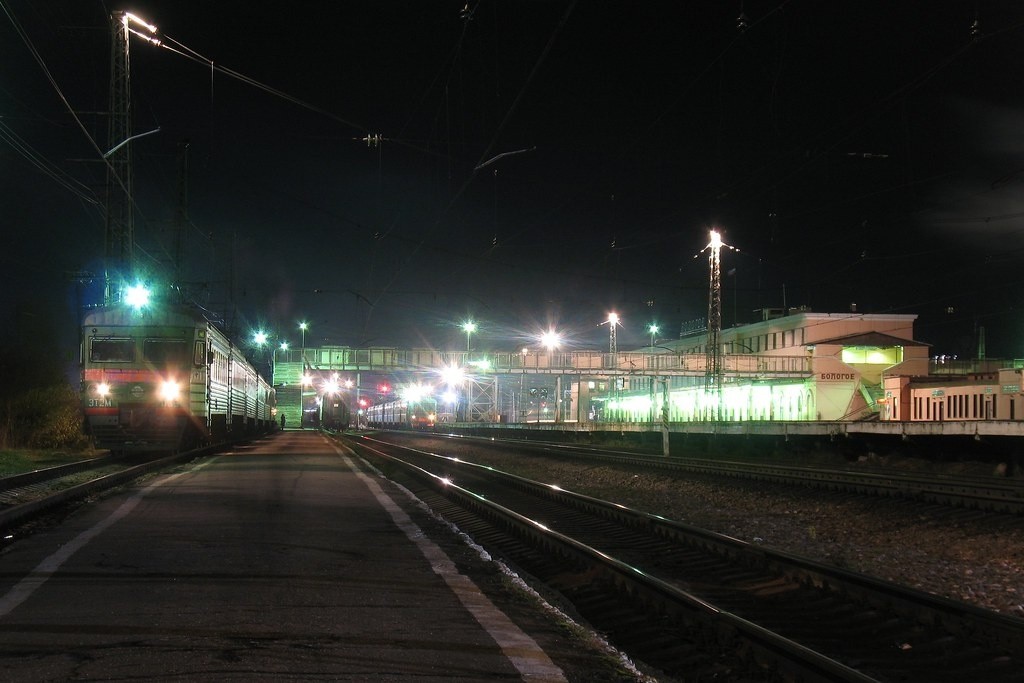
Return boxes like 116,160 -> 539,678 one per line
80,302 -> 277,446
320,390 -> 350,429
368,397 -> 438,428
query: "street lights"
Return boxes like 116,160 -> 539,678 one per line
650,324 -> 657,353
543,331 -> 559,373
464,322 -> 475,351
711,232 -> 721,376
609,313 -> 620,366
380,383 -> 388,429
300,322 -> 307,348
